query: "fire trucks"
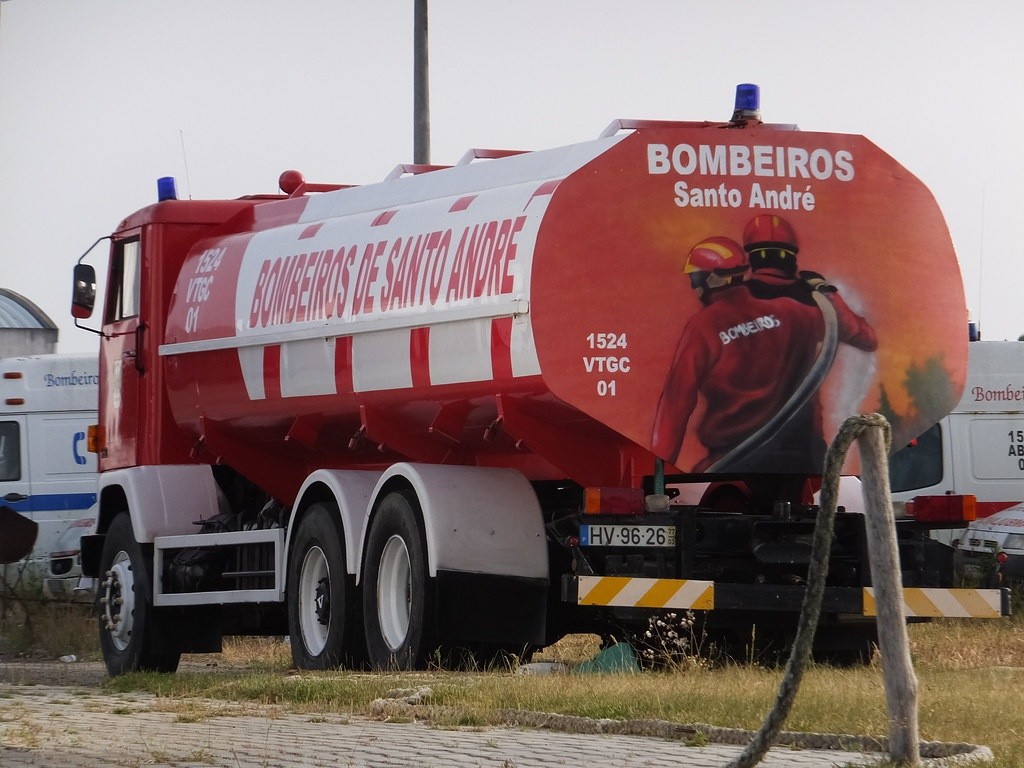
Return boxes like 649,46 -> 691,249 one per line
71,85 -> 970,681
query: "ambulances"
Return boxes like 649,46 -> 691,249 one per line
888,325 -> 1023,585
0,357 -> 98,602
